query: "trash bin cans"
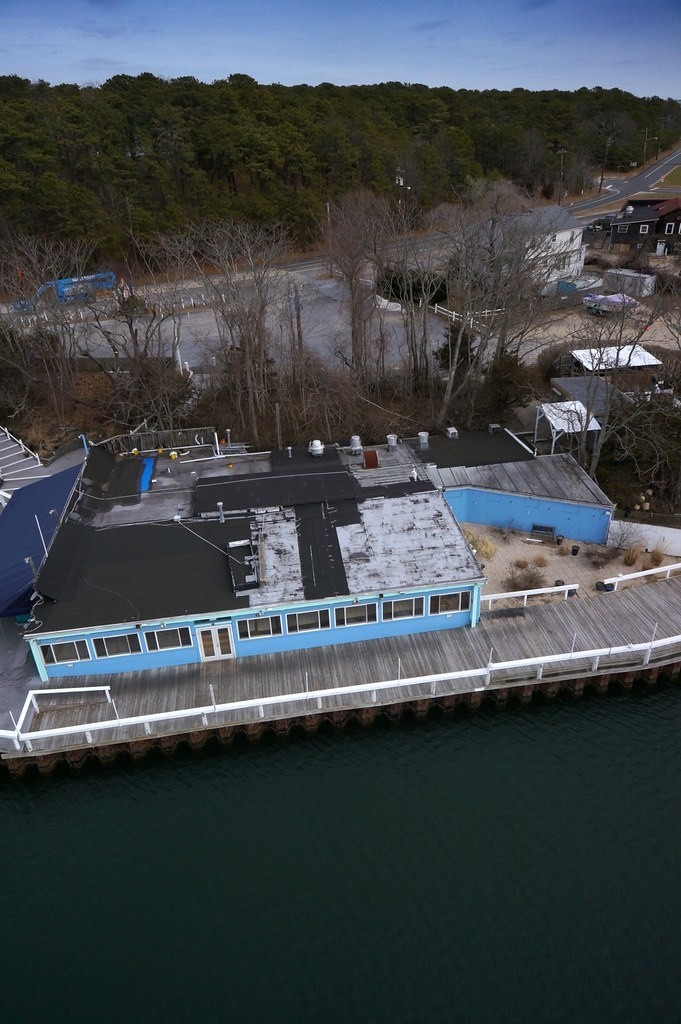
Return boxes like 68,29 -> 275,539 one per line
555,580 -> 564,586
571,545 -> 580,556
556,535 -> 564,545
596,582 -> 614,592
568,589 -> 576,596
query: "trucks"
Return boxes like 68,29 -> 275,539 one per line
27,272 -> 116,309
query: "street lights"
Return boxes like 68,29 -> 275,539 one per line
598,138 -> 612,194
642,128 -> 658,169
555,149 -> 568,206
395,167 -> 410,238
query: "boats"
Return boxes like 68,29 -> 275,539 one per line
583,292 -> 641,313
